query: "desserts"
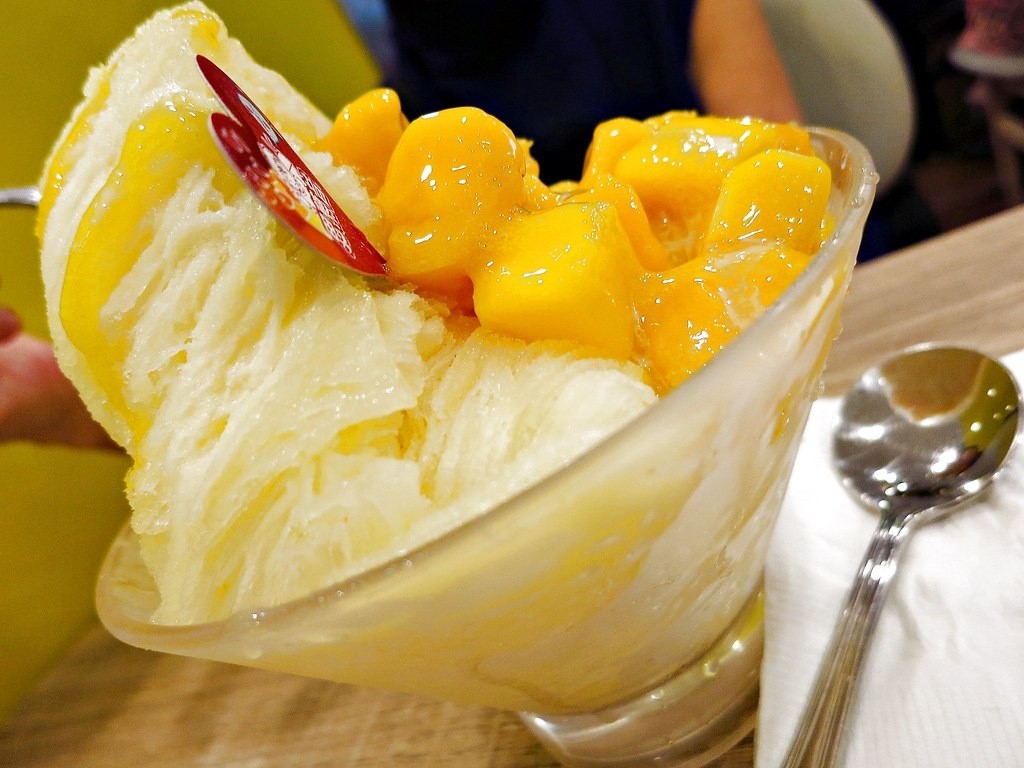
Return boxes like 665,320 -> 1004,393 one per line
28,0 -> 838,719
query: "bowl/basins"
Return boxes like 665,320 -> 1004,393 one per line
92,120 -> 878,768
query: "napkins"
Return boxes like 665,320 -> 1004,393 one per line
753,348 -> 1023,768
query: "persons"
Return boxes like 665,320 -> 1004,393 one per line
382,0 -> 806,189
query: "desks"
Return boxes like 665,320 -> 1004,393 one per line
0,203 -> 1023,768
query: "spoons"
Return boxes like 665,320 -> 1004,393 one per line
778,347 -> 1018,768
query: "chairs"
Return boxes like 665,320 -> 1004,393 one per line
757,0 -> 915,194
